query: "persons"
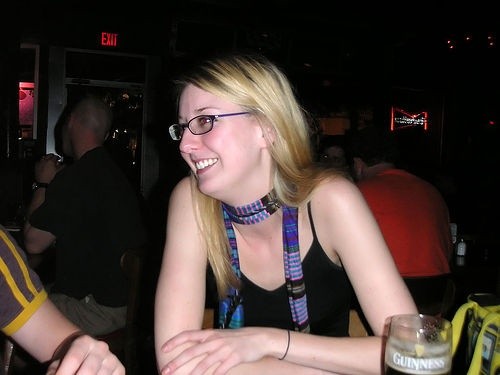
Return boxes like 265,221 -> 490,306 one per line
346,126 -> 454,322
153,49 -> 450,375
13,92 -> 139,370
0,223 -> 126,375
317,137 -> 351,172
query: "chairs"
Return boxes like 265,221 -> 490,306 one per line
93,249 -> 162,375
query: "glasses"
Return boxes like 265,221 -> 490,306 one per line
168,112 -> 252,141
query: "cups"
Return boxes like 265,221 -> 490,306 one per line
385,315 -> 453,375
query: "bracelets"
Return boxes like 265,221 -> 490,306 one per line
278,329 -> 290,360
